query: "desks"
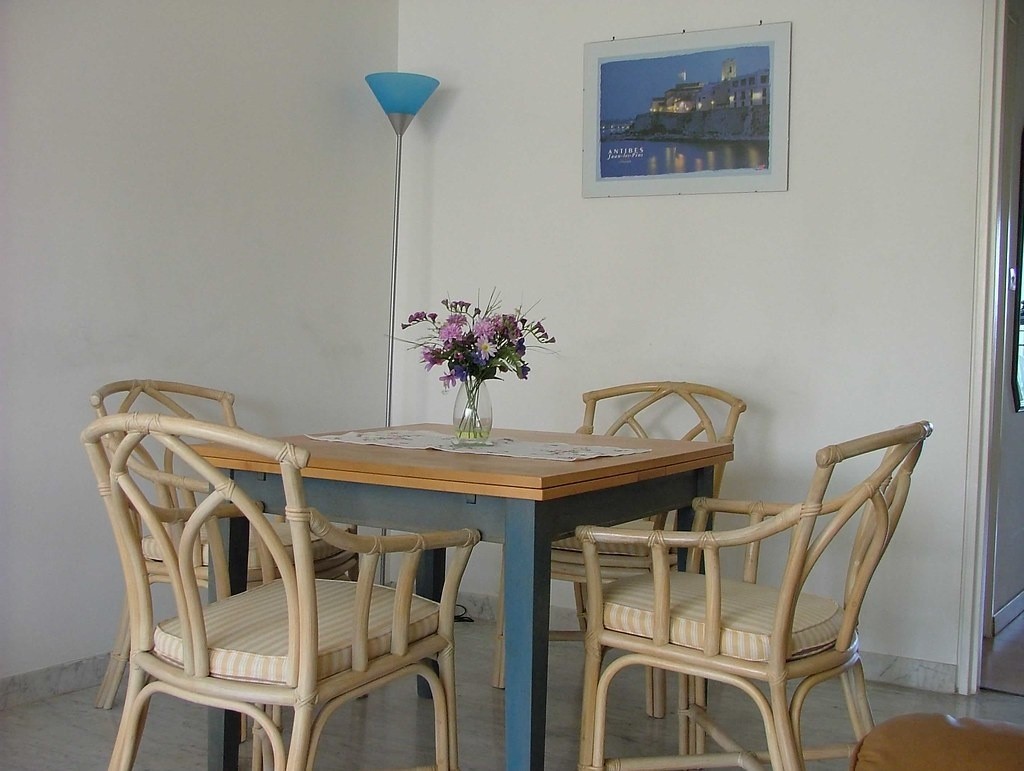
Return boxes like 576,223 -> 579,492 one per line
183,424 -> 735,771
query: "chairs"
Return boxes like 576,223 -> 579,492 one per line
574,419 -> 936,771
490,379 -> 746,721
89,377 -> 362,744
77,413 -> 483,771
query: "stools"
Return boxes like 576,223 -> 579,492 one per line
848,712 -> 1024,771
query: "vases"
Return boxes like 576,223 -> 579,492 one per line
452,380 -> 494,446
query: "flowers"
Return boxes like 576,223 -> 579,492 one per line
382,282 -> 560,444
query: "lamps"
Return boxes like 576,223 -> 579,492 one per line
363,71 -> 442,425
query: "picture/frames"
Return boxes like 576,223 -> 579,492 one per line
579,22 -> 791,203
